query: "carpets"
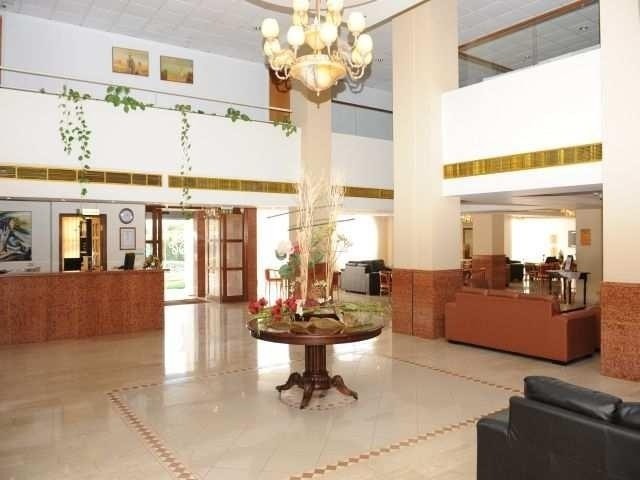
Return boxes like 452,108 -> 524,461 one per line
105,347 -> 526,480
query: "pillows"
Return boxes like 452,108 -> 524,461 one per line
525,372 -> 640,428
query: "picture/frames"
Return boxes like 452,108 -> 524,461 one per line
120,228 -> 136,249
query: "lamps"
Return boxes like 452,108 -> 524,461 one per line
260,0 -> 373,97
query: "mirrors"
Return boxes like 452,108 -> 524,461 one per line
462,228 -> 473,260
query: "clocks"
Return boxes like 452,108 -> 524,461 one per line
119,208 -> 134,223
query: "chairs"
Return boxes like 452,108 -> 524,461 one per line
505,257 -> 524,281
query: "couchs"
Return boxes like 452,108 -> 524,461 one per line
446,287 -> 595,363
478,395 -> 640,480
341,259 -> 392,296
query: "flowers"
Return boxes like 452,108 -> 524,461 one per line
245,296 -> 317,322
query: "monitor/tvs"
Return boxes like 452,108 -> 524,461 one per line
124,253 -> 135,270
64,258 -> 81,272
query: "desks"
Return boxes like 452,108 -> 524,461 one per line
245,306 -> 385,410
543,269 -> 591,310
